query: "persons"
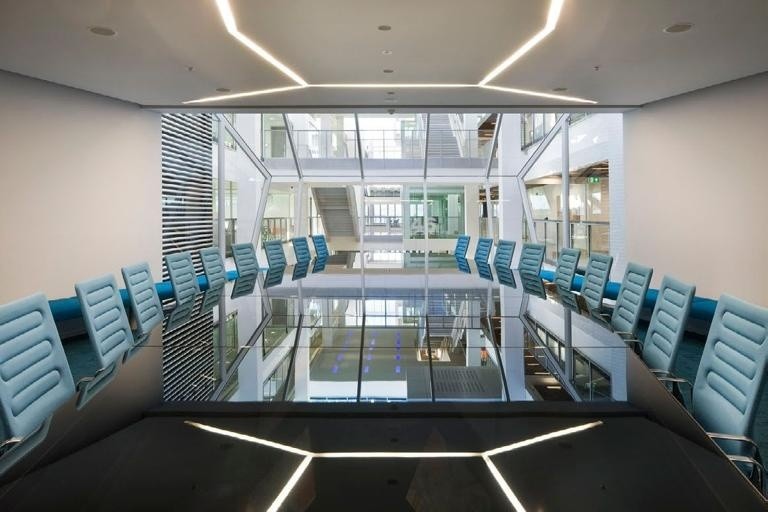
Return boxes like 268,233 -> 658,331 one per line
480,347 -> 488,368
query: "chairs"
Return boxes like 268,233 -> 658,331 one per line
313,256 -> 329,274
0,291 -> 78,455
474,260 -> 494,282
122,331 -> 152,367
310,234 -> 329,258
264,268 -> 287,289
231,271 -> 260,299
556,283 -> 582,315
163,251 -> 198,305
199,247 -> 229,290
290,236 -> 312,262
493,238 -> 517,269
1,410 -> 56,475
518,270 -> 548,300
76,354 -> 120,413
120,260 -> 165,334
262,239 -> 288,268
73,273 -> 135,365
474,236 -> 494,262
454,233 -> 472,258
581,297 -> 614,333
493,265 -> 517,289
517,240 -> 546,276
455,256 -> 472,274
578,251 -> 614,311
163,292 -> 198,335
199,282 -> 226,316
231,242 -> 261,276
654,293 -> 767,498
544,245 -> 581,292
292,261 -> 311,281
591,259 -> 654,348
619,273 -> 698,409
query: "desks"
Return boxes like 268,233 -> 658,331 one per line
0,270 -> 768,511
